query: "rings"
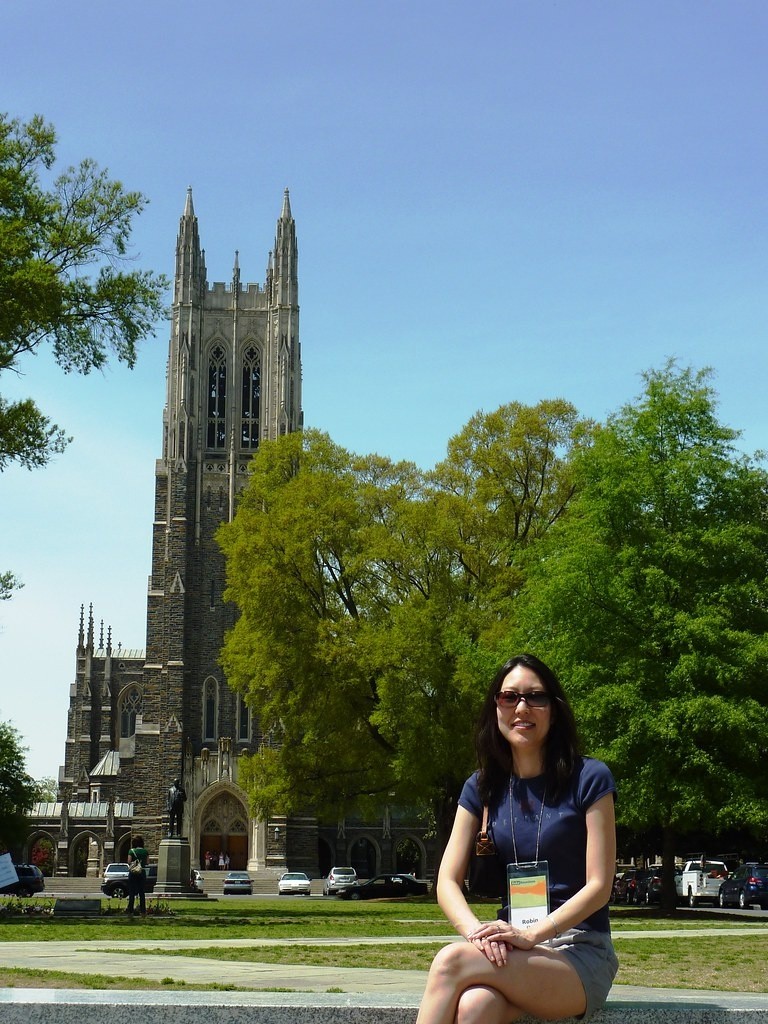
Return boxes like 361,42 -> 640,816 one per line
496,925 -> 500,933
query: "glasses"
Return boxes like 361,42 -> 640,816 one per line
494,691 -> 552,708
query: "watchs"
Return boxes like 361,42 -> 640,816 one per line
546,915 -> 563,940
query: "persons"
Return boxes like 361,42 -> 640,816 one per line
125,835 -> 151,916
410,650 -> 620,1023
167,778 -> 188,838
204,849 -> 232,871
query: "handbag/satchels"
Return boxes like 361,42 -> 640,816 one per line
128,849 -> 143,873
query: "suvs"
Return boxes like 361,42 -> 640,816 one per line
323,866 -> 361,894
102,861 -> 196,898
0,863 -> 46,898
102,863 -> 130,883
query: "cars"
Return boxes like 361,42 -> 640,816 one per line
276,872 -> 314,895
222,873 -> 254,896
336,875 -> 427,900
392,874 -> 417,883
607,855 -> 736,905
192,870 -> 204,893
718,862 -> 767,909
